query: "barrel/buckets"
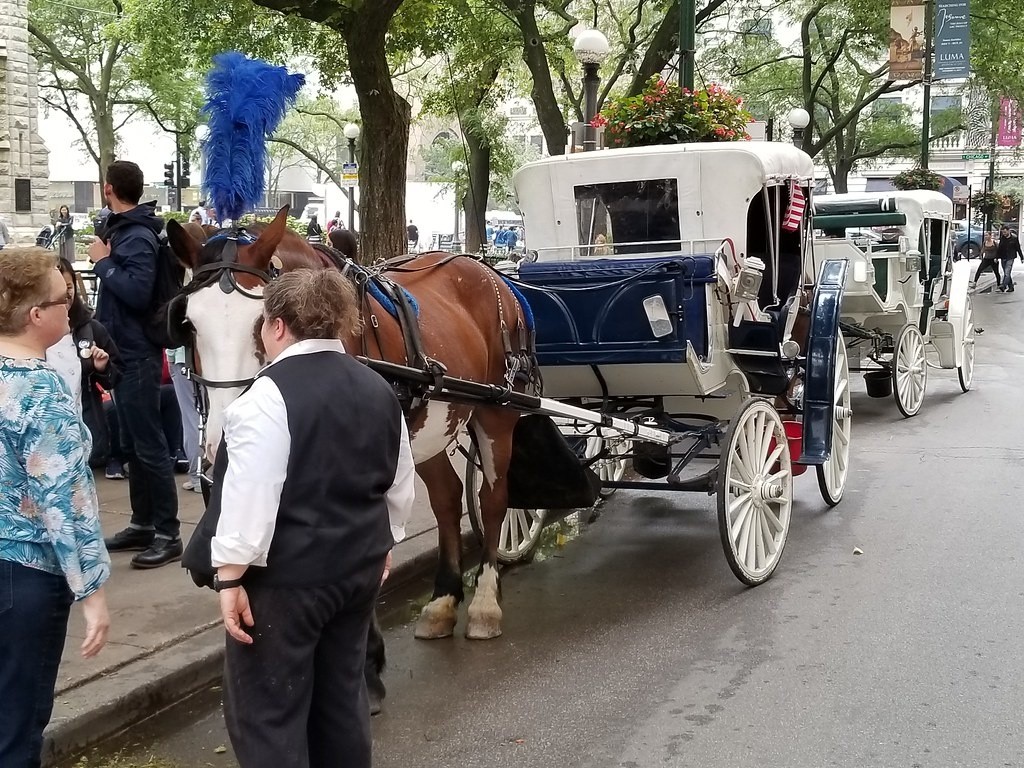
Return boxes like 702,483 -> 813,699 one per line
765,420 -> 807,478
862,357 -> 893,399
632,440 -> 672,479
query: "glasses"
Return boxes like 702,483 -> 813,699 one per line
192,220 -> 196,223
39,294 -> 73,311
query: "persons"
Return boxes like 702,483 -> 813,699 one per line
994,226 -> 1024,292
87,162 -> 184,568
307,211 -> 346,247
485,221 -> 524,255
54,205 -> 77,265
969,231 -> 1001,292
0,246 -> 111,768
189,198 -> 233,228
210,267 -> 415,768
0,223 -> 218,493
407,220 -> 419,250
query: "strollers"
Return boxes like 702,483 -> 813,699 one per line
35,224 -> 67,250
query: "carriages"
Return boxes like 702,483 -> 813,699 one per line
165,143 -> 853,638
791,188 -> 979,417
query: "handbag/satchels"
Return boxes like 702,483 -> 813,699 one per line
309,234 -> 321,245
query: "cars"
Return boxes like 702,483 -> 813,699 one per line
952,219 -> 986,259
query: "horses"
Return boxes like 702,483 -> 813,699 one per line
791,272 -> 815,376
166,204 -> 536,713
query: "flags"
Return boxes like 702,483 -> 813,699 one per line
782,178 -> 806,232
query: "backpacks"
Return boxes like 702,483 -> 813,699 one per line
148,227 -> 185,349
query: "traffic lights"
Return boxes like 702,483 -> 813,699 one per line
165,163 -> 174,187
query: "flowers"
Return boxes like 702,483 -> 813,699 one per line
972,191 -> 1004,213
888,169 -> 945,190
590,73 -> 756,148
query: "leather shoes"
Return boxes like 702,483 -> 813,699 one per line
103,528 -> 156,552
130,539 -> 183,568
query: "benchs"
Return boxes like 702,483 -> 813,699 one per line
919,255 -> 948,310
515,254 -> 730,395
746,253 -> 802,341
872,256 -> 902,310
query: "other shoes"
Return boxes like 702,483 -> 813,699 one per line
175,449 -> 189,473
1006,287 -> 1014,292
995,288 -> 1002,292
969,284 -> 976,289
105,457 -> 125,478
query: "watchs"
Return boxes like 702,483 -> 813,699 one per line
213,574 -> 241,592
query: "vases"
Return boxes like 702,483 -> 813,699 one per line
980,204 -> 995,213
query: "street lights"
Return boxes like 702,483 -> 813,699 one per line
574,29 -> 611,151
343,123 -> 361,233
789,109 -> 810,150
195,125 -> 211,208
